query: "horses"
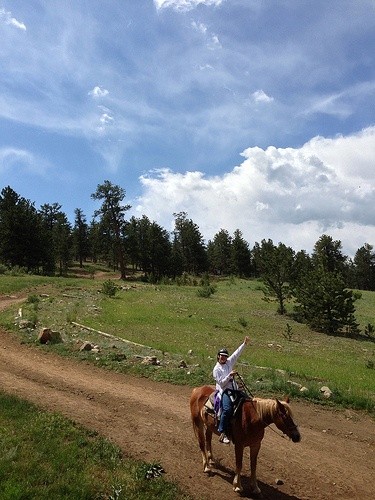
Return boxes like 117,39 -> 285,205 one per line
190,386 -> 301,499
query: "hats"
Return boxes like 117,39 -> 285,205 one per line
217,348 -> 229,357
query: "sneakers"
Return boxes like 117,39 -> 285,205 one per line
222,436 -> 230,444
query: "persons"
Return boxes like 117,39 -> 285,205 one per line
212,335 -> 251,444
